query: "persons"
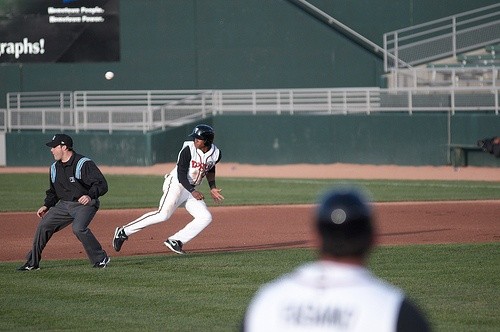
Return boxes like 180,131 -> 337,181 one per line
238,185 -> 435,332
16,133 -> 112,272
110,123 -> 226,255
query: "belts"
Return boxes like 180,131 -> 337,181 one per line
190,184 -> 196,187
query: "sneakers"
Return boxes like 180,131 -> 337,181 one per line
93,256 -> 111,268
163,240 -> 185,254
16,263 -> 40,271
112,227 -> 128,252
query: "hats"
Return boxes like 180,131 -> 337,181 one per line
45,134 -> 73,148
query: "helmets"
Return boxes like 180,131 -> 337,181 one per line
187,124 -> 214,146
315,191 -> 374,255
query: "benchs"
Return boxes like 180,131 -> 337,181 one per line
449,145 -> 486,172
427,46 -> 500,86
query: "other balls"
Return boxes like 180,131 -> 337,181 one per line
104,70 -> 114,80
